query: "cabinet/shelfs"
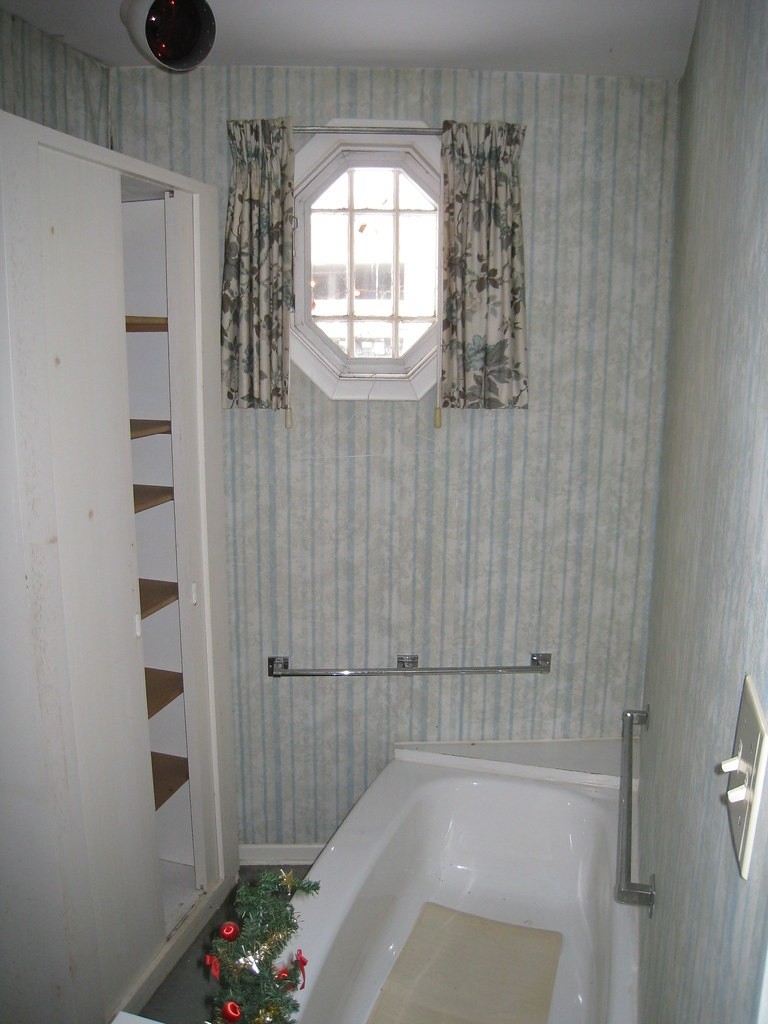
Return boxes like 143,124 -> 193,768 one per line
0,110 -> 241,1024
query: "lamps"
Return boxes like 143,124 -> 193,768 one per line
119,0 -> 219,74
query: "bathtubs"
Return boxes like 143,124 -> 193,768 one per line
244,757 -> 615,1023
